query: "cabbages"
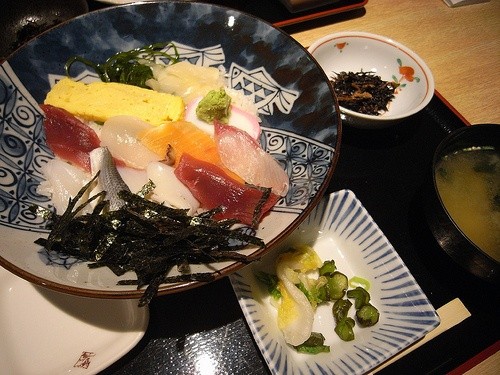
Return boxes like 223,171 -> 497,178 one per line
255,234 -> 330,353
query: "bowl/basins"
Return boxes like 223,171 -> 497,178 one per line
307,30 -> 435,125
421,124 -> 500,284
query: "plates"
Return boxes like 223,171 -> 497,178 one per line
0,0 -> 343,301
228,189 -> 441,375
0,266 -> 150,375
